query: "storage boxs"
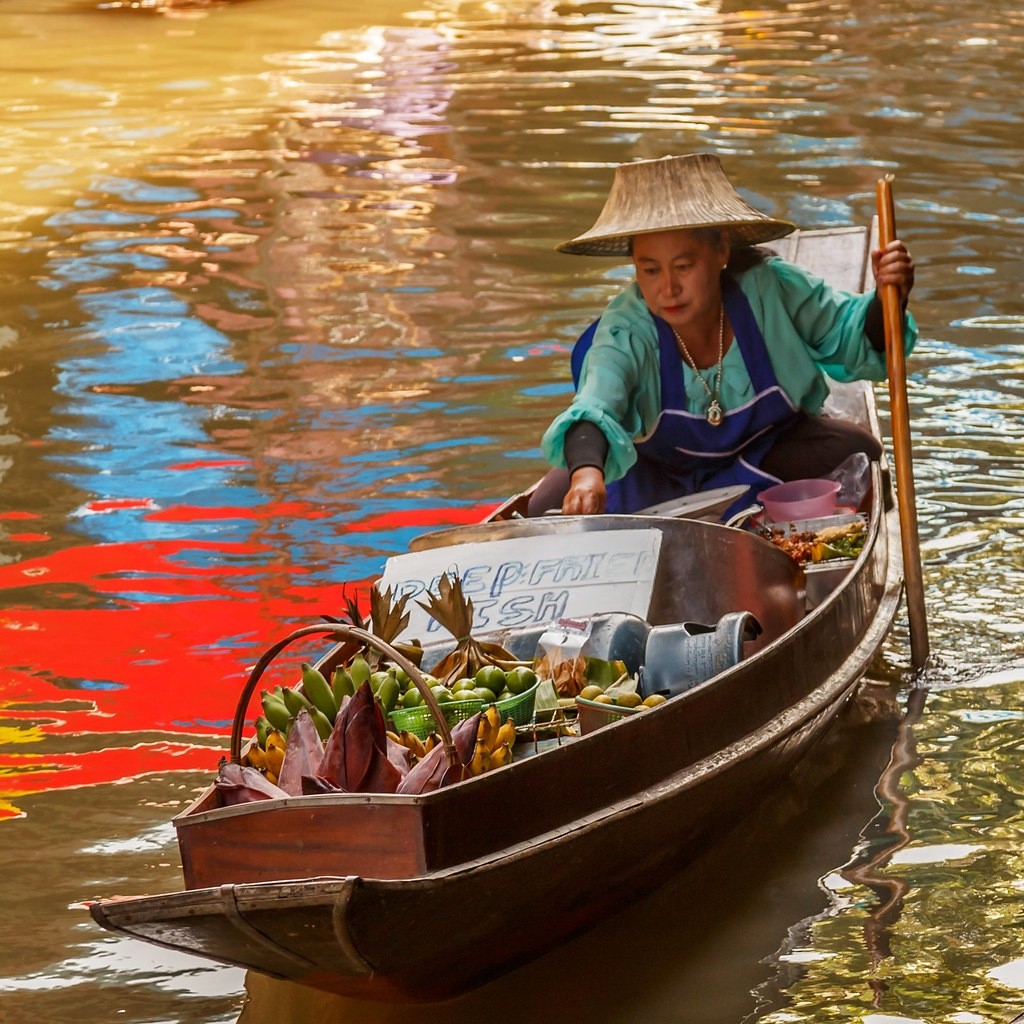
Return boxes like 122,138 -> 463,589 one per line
758,512 -> 867,611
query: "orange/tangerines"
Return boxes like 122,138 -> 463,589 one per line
579,684 -> 667,712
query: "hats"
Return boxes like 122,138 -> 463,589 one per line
554,153 -> 796,257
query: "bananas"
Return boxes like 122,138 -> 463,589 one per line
247,652 -> 517,775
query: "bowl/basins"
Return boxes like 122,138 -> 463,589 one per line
755,479 -> 842,523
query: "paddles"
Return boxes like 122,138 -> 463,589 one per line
875,175 -> 929,665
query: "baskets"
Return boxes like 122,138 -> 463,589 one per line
469,672 -> 541,726
216,623 -> 472,807
575,695 -> 644,736
749,514 -> 867,563
388,699 -> 485,743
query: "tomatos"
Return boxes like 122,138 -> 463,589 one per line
402,664 -> 536,703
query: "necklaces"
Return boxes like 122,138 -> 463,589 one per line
672,301 -> 724,426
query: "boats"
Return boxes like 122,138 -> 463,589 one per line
86,213 -> 910,1005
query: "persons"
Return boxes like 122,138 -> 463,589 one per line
527,154 -> 921,528
